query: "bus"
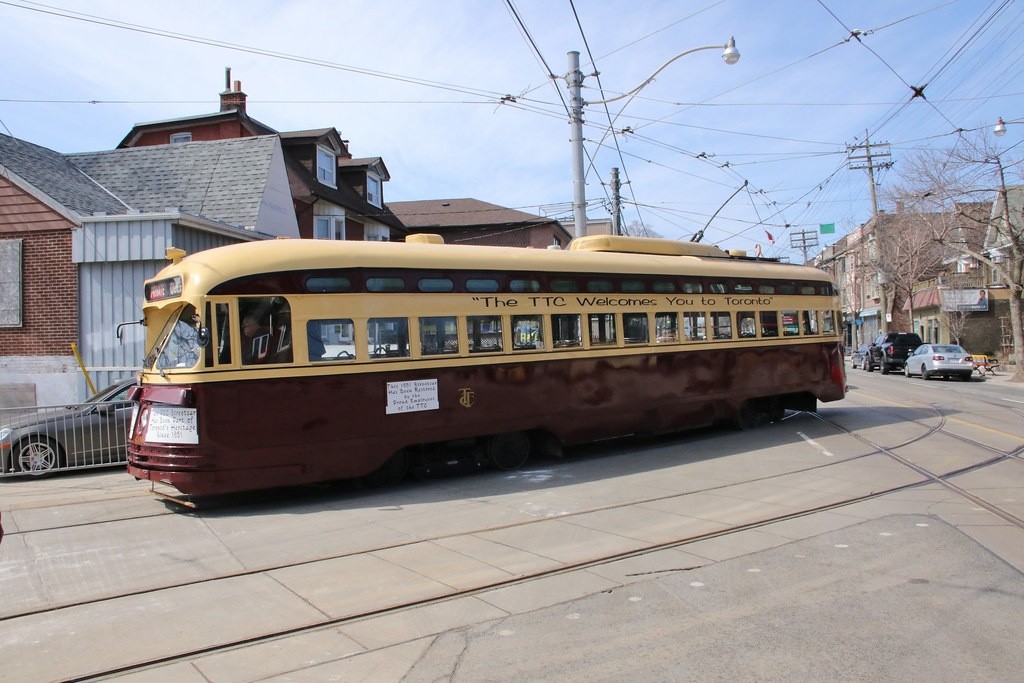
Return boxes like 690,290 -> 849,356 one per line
112,232 -> 847,500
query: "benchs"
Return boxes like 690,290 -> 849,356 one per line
972,354 -> 1002,377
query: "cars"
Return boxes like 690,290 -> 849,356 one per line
850,343 -> 872,370
903,343 -> 974,381
0,378 -> 138,480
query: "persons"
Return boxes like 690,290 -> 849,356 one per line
976,290 -> 987,304
241,316 -> 271,362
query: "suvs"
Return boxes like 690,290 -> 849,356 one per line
864,332 -> 923,375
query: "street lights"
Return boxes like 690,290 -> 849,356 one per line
566,38 -> 742,234
